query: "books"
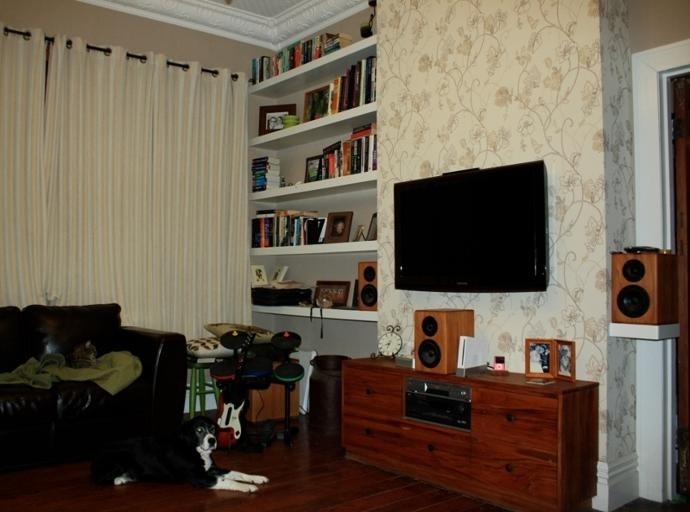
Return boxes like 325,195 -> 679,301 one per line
251,32 -> 377,248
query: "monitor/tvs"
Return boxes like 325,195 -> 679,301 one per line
393,159 -> 550,294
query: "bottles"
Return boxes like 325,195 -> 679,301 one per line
494,355 -> 505,371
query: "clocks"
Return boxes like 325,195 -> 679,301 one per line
371,325 -> 402,360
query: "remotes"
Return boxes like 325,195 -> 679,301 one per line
624,246 -> 659,254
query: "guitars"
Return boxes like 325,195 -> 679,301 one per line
215,334 -> 256,451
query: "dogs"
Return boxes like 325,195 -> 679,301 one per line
89,415 -> 269,493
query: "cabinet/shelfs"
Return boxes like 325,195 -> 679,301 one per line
341,356 -> 599,512
246,35 -> 377,320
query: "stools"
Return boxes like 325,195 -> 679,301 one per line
187,358 -> 221,419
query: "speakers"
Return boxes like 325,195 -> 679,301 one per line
358,262 -> 376,311
413,308 -> 473,376
610,252 -> 679,325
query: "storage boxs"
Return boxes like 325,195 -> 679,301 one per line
244,357 -> 299,422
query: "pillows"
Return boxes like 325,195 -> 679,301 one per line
187,336 -> 242,358
204,323 -> 275,344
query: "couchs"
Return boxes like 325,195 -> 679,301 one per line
1,303 -> 187,475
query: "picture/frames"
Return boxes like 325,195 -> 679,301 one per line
525,339 -> 557,378
555,340 -> 575,382
251,265 -> 268,285
304,155 -> 324,183
314,281 -> 351,307
259,105 -> 296,135
303,86 -> 332,122
324,211 -> 353,243
